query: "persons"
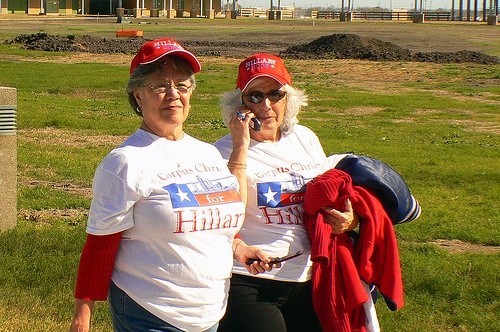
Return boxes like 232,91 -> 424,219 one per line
212,52 -> 360,332
69,37 -> 281,332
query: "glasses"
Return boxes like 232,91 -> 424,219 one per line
243,89 -> 288,104
142,80 -> 195,92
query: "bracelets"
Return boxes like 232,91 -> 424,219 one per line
226,160 -> 248,170
233,239 -> 245,262
347,221 -> 361,236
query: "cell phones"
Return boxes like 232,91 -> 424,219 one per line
249,117 -> 261,132
245,249 -> 305,265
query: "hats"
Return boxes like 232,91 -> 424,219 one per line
235,54 -> 292,92
130,38 -> 201,76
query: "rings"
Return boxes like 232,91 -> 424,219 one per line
332,219 -> 339,224
236,111 -> 246,122
